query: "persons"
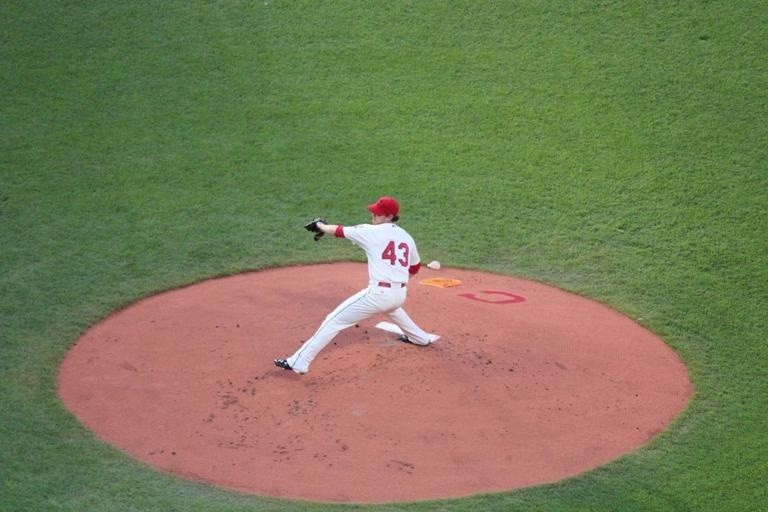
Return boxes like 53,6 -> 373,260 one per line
272,195 -> 432,374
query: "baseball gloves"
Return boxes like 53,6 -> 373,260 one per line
304,217 -> 328,242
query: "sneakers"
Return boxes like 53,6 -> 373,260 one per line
274,359 -> 293,370
400,334 -> 412,343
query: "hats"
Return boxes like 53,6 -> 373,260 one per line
366,196 -> 400,216
